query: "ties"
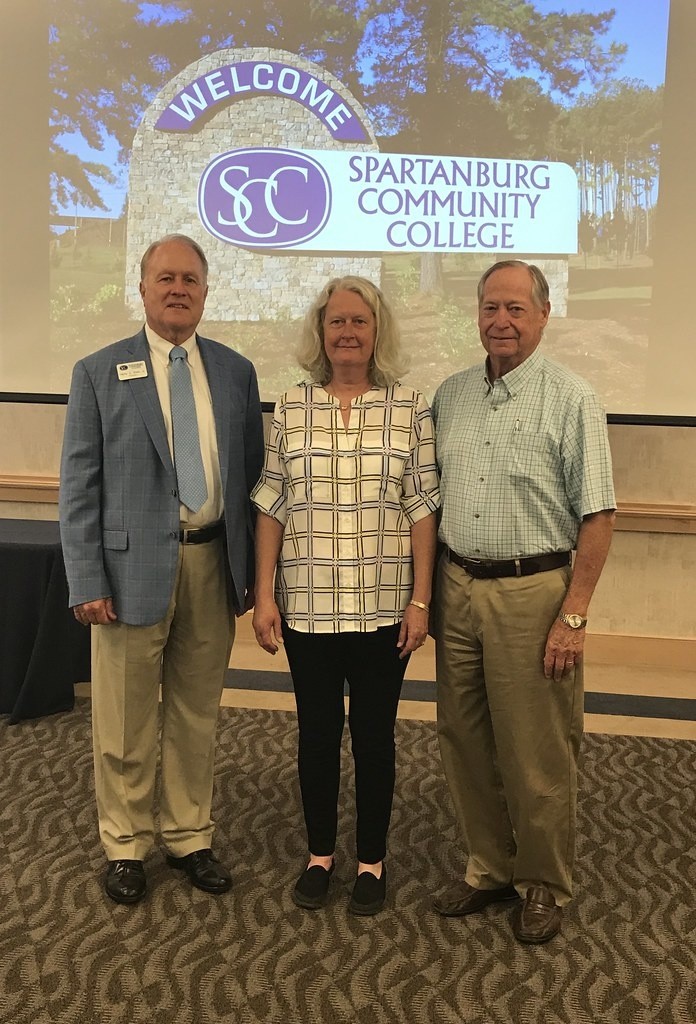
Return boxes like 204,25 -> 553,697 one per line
170,347 -> 208,514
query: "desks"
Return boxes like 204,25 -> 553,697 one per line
0,518 -> 92,726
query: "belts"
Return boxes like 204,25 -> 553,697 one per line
443,543 -> 571,580
179,523 -> 225,545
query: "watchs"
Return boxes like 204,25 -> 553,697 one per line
557,612 -> 591,633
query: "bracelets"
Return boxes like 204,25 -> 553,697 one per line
409,598 -> 431,615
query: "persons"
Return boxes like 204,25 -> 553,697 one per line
250,275 -> 442,917
57,232 -> 264,903
429,259 -> 618,946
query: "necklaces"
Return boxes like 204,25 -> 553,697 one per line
326,379 -> 371,411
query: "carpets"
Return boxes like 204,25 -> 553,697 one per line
0,696 -> 696,1024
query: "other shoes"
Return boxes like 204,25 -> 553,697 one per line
348,860 -> 387,915
292,857 -> 336,909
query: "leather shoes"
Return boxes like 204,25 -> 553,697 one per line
166,849 -> 231,892
106,860 -> 147,902
432,881 -> 519,916
514,887 -> 563,943
